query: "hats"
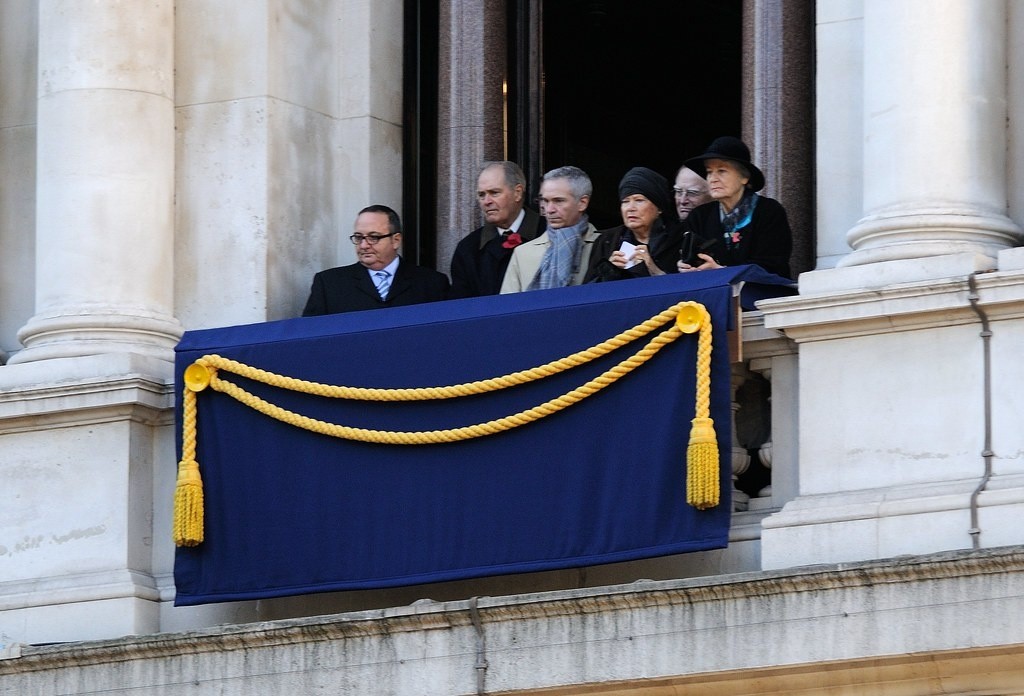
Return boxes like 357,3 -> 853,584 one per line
683,137 -> 765,193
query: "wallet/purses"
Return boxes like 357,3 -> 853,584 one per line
681,232 -> 718,268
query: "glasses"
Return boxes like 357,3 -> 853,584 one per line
350,231 -> 402,245
533,197 -> 544,204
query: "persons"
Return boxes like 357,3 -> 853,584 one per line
450,160 -> 547,299
500,166 -> 601,296
673,165 -> 717,224
663,138 -> 792,279
581,167 -> 683,286
302,205 -> 451,318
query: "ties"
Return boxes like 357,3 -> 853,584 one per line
374,271 -> 394,301
501,230 -> 513,241
